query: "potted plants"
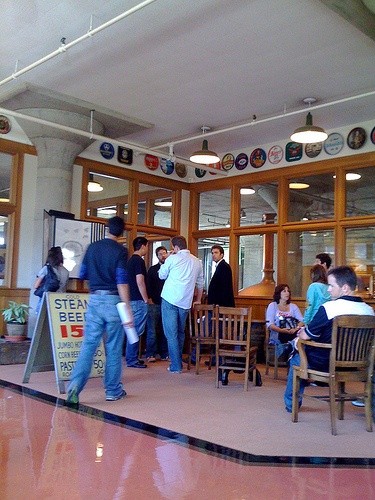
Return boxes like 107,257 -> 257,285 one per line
0,300 -> 33,336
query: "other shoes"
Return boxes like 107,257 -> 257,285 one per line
352,400 -> 366,407
166,366 -> 182,374
106,390 -> 125,401
67,387 -> 79,405
182,358 -> 197,364
205,361 -> 219,366
136,359 -> 144,364
147,355 -> 155,362
154,354 -> 168,360
127,363 -> 146,368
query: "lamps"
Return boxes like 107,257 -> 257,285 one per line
189,125 -> 221,164
289,96 -> 328,143
87,174 -> 104,192
290,178 -> 310,189
240,186 -> 256,195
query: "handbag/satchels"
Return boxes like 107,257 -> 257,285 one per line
34,265 -> 60,298
279,316 -> 300,343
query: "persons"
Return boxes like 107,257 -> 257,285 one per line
147,247 -> 173,362
33,246 -> 70,318
266,284 -> 303,367
159,236 -> 204,374
204,245 -> 235,367
120,236 -> 153,368
63,216 -> 133,404
313,253 -> 332,276
284,266 -> 375,412
298,264 -> 334,330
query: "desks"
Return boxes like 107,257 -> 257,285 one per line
210,316 -> 266,363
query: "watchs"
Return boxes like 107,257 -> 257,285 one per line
296,327 -> 300,329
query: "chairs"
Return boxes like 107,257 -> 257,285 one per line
292,314 -> 375,436
187,303 -> 219,375
265,307 -> 289,378
215,305 -> 258,392
